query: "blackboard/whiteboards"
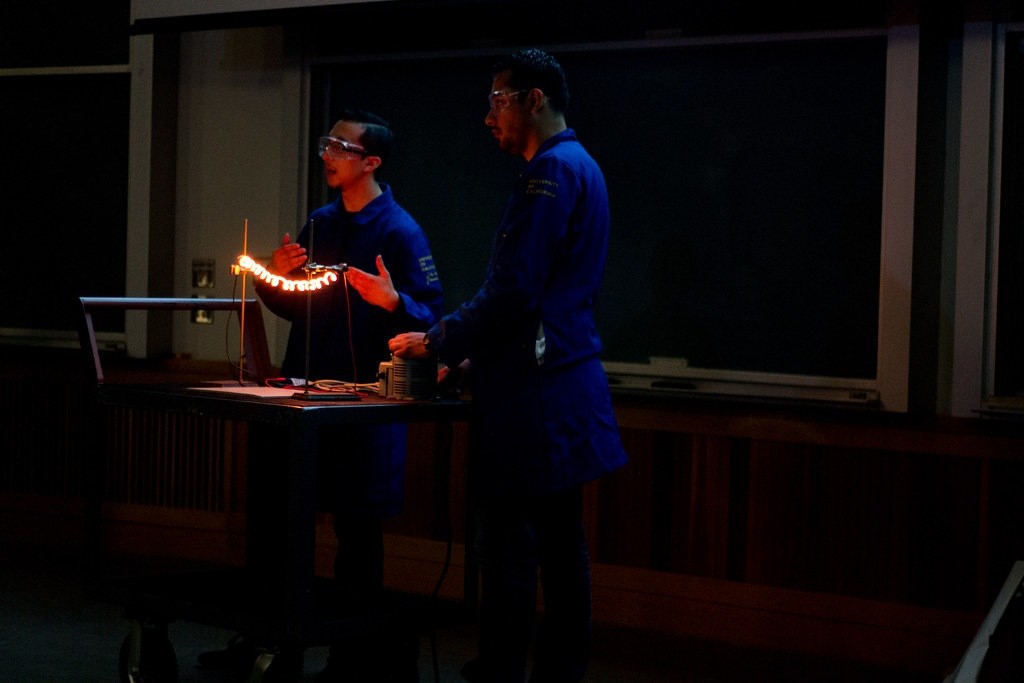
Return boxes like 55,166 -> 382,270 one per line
302,19 -> 924,413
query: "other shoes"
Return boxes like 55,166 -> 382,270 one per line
528,662 -> 589,682
462,655 -> 527,683
196,639 -> 304,675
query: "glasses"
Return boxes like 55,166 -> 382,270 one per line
488,88 -> 532,112
317,136 -> 368,159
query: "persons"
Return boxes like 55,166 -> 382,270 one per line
194,105 -> 447,683
386,45 -> 631,680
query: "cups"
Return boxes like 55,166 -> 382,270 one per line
394,354 -> 438,400
